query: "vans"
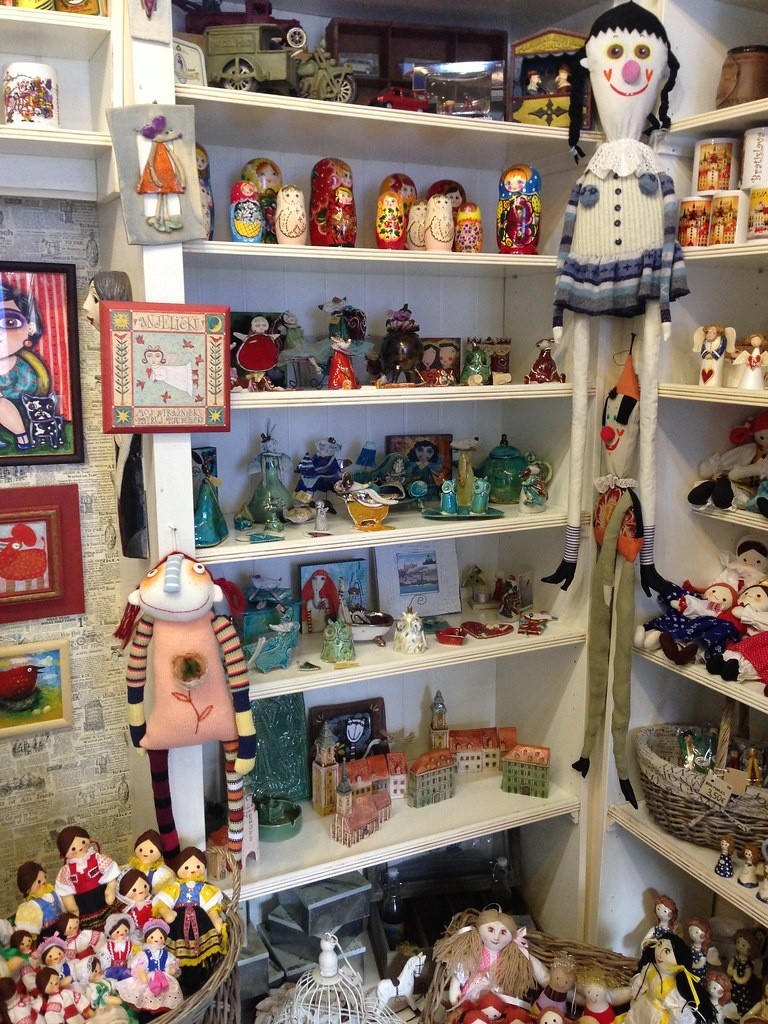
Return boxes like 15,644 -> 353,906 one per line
371,84 -> 429,113
342,59 -> 375,75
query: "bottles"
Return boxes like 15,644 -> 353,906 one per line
380,868 -> 408,952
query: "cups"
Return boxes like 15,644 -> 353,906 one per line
3,61 -> 60,131
675,126 -> 767,252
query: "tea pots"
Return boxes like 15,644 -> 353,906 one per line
473,434 -> 553,504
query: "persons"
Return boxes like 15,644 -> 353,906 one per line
571,333 -> 641,811
756,862 -> 768,904
685,916 -> 708,987
686,412 -> 768,519
435,907 -> 718,1023
714,837 -> 734,878
117,553 -> 258,872
634,533 -> 768,698
726,745 -> 761,788
0,826 -> 228,1024
738,843 -> 761,888
83,271 -> 152,560
541,1 -> 690,598
726,928 -> 755,1017
697,323 -> 727,387
639,895 -> 678,947
741,977 -> 768,1023
526,71 -> 542,95
281,310 -> 331,375
739,333 -> 767,390
555,64 -> 572,94
314,501 -> 329,531
706,970 -> 732,1024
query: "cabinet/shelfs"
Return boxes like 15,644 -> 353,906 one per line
122,0 -> 601,944
585,0 -> 768,1005
0,0 -> 120,204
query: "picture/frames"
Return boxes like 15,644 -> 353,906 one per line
0,638 -> 72,738
309,695 -> 389,759
371,540 -> 463,619
0,482 -> 86,624
0,256 -> 85,467
100,301 -> 232,435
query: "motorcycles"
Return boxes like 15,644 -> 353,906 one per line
202,21 -> 359,107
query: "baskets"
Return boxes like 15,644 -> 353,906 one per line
418,908 -> 642,1024
117,845 -> 246,1024
634,723 -> 768,858
